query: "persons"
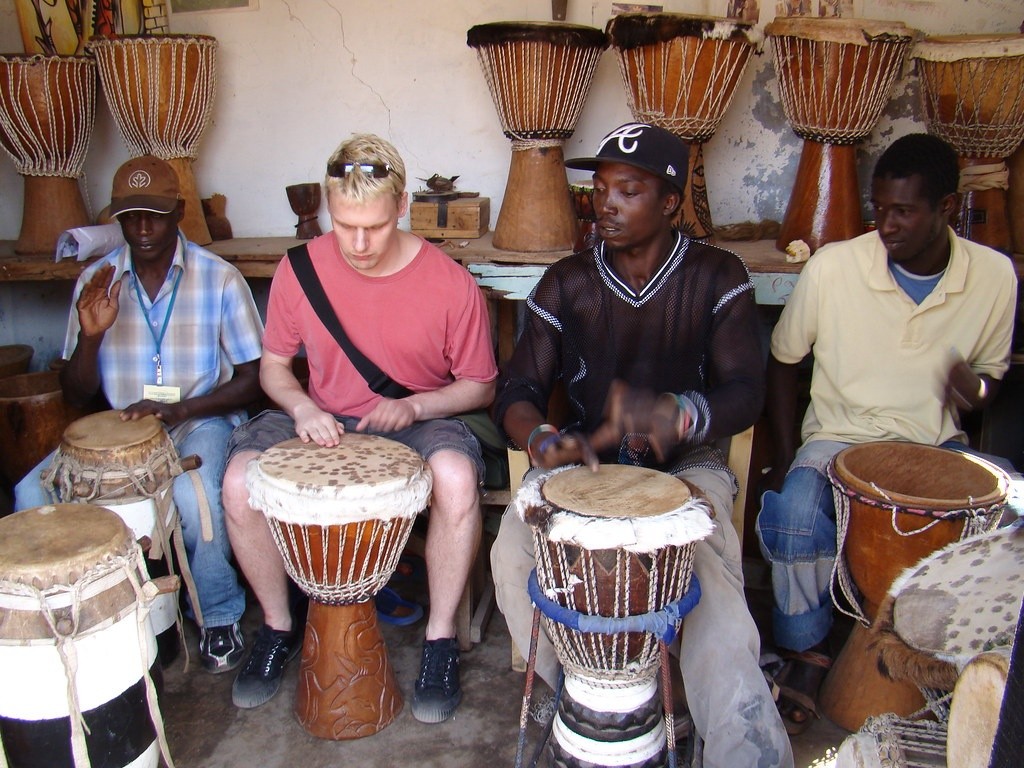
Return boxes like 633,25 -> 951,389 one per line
11,153 -> 264,676
756,131 -> 1019,735
485,120 -> 799,768
217,133 -> 502,722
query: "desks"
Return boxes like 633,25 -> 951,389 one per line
0,237 -> 811,304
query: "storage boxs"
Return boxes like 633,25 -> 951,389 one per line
410,197 -> 490,239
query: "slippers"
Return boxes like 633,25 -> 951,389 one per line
372,586 -> 422,625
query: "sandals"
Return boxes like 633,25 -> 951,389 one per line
774,650 -> 833,735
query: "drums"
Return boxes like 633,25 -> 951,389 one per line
538,463 -> 696,768
1,53 -> 100,255
815,440 -> 1013,732
257,433 -> 426,741
62,407 -> 178,550
468,22 -> 604,252
920,34 -> 1023,256
85,35 -> 216,247
773,15 -> 906,253
0,503 -> 162,768
612,14 -> 758,241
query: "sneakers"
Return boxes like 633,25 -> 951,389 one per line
232,623 -> 305,708
410,636 -> 462,723
198,622 -> 244,673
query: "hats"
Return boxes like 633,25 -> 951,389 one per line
108,152 -> 181,217
564,123 -> 689,193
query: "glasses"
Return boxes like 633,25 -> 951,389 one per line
327,161 -> 402,181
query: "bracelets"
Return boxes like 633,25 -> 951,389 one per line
525,424 -> 559,461
664,388 -> 711,444
